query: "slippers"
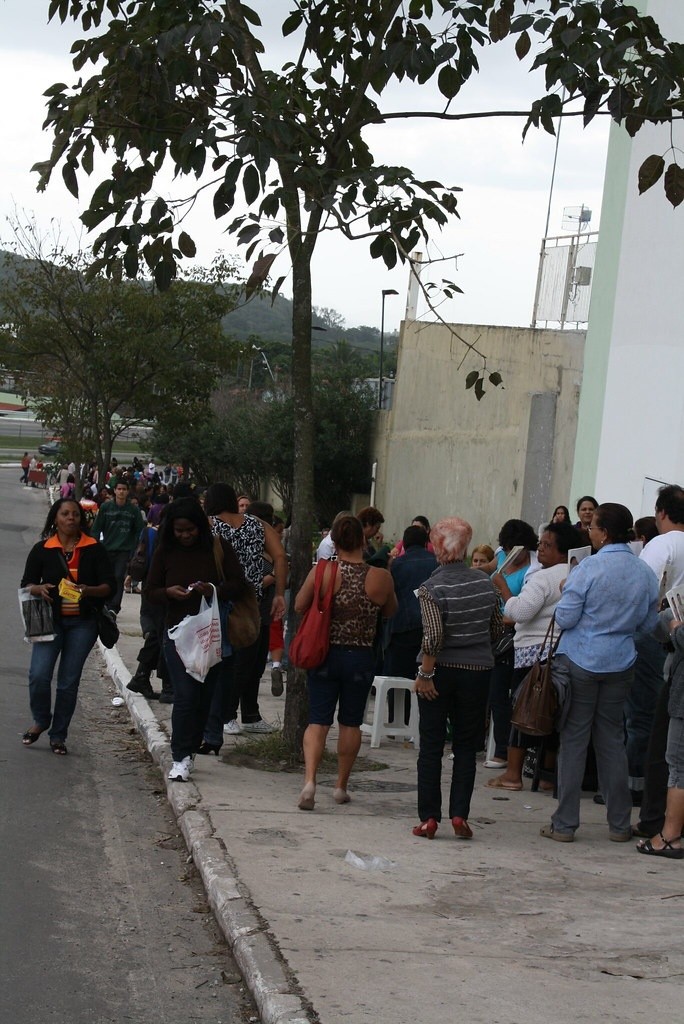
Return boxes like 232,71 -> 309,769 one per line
483,778 -> 518,791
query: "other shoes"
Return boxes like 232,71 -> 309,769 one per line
270,668 -> 285,695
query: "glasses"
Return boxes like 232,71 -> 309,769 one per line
537,542 -> 560,548
587,526 -> 604,532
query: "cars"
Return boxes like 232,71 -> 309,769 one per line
38,440 -> 66,457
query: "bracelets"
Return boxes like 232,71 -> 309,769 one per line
417,665 -> 435,680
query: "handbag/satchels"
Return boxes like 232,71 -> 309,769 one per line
509,609 -> 571,736
98,604 -> 119,648
167,583 -> 223,683
213,534 -> 261,649
128,549 -> 149,581
290,558 -> 340,670
18,587 -> 58,644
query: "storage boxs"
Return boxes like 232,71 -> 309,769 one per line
58,578 -> 81,604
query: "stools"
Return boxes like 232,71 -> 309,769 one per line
360,676 -> 420,750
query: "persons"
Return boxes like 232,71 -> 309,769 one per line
78,481 -> 208,594
19,452 -> 38,488
295,516 -> 400,810
19,498 -> 118,754
315,506 -> 495,759
123,483 -> 288,781
471,485 -> 684,860
89,481 -> 143,622
55,456 -> 184,500
412,518 -> 494,840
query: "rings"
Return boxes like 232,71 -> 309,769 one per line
418,692 -> 423,696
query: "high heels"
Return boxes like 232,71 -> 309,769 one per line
197,739 -> 224,755
297,781 -> 316,809
413,819 -> 439,839
452,817 -> 474,836
333,788 -> 351,803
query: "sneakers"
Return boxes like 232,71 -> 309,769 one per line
127,676 -> 161,699
224,719 -> 243,735
160,689 -> 174,702
168,755 -> 196,781
244,720 -> 278,733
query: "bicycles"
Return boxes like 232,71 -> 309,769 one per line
49,461 -> 62,485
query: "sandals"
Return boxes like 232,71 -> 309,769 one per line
540,784 -> 684,858
50,741 -> 68,754
22,720 -> 50,743
483,760 -> 510,768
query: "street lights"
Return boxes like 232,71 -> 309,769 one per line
379,289 -> 400,410
